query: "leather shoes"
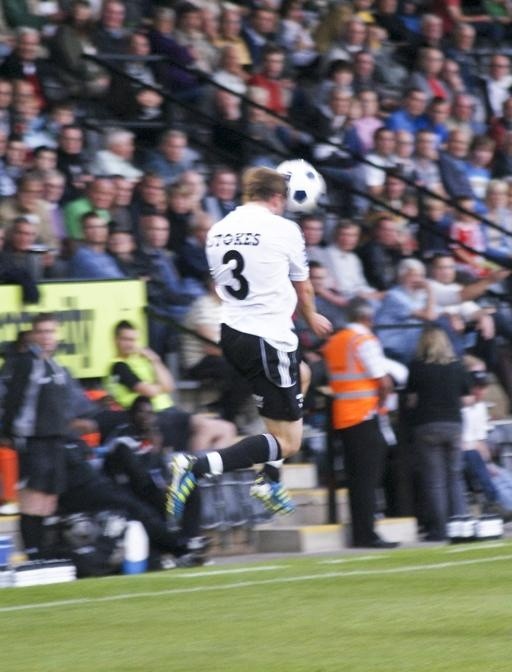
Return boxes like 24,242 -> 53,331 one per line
353,536 -> 401,549
422,531 -> 448,540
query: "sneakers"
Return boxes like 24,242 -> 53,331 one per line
250,475 -> 297,515
166,452 -> 197,534
0,515 -> 214,578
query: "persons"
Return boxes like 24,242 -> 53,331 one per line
0,0 -> 512,580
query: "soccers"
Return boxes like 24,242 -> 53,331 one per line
274,157 -> 327,216
